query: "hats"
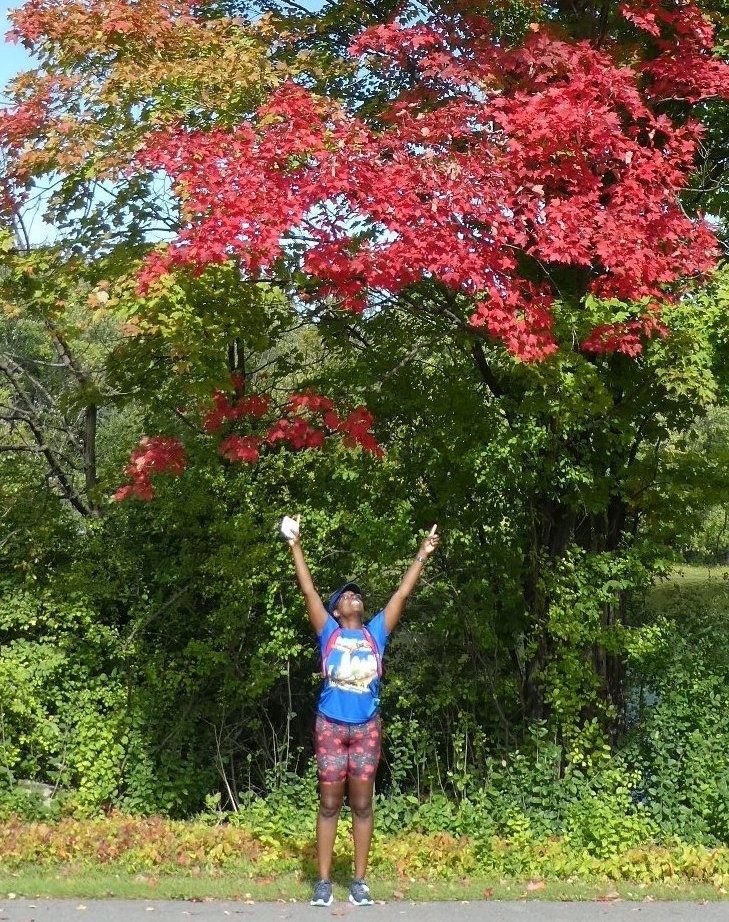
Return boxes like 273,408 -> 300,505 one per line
329,583 -> 361,616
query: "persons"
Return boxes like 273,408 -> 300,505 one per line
281,513 -> 440,908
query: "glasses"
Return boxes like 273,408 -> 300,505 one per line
335,592 -> 361,610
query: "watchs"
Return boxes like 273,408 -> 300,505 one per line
414,554 -> 428,564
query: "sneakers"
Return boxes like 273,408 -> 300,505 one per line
310,880 -> 334,906
348,880 -> 374,906
